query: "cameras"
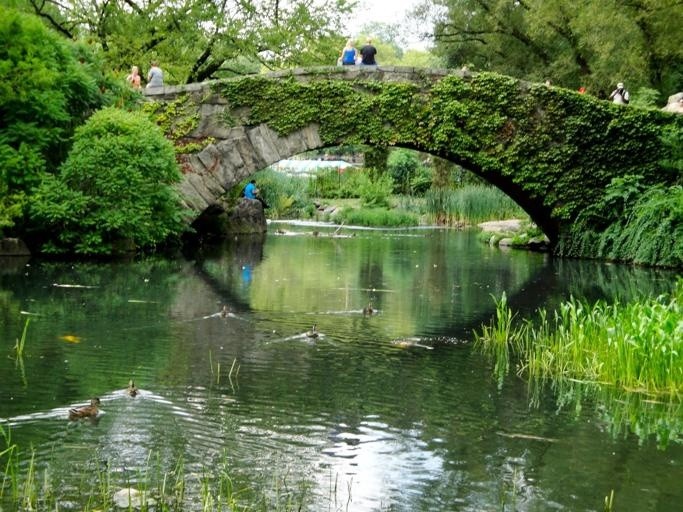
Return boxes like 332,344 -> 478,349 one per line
618,89 -> 623,94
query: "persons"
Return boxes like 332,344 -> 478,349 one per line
337,50 -> 343,65
545,79 -> 553,85
340,40 -> 358,65
667,92 -> 683,112
359,40 -> 376,64
598,90 -> 606,100
126,65 -> 141,90
608,82 -> 630,105
146,60 -> 163,88
243,180 -> 271,209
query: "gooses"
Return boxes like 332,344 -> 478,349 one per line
363,301 -> 374,313
127,378 -> 138,396
307,323 -> 318,338
68,397 -> 102,416
221,305 -> 226,318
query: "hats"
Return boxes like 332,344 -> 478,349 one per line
616,82 -> 624,89
